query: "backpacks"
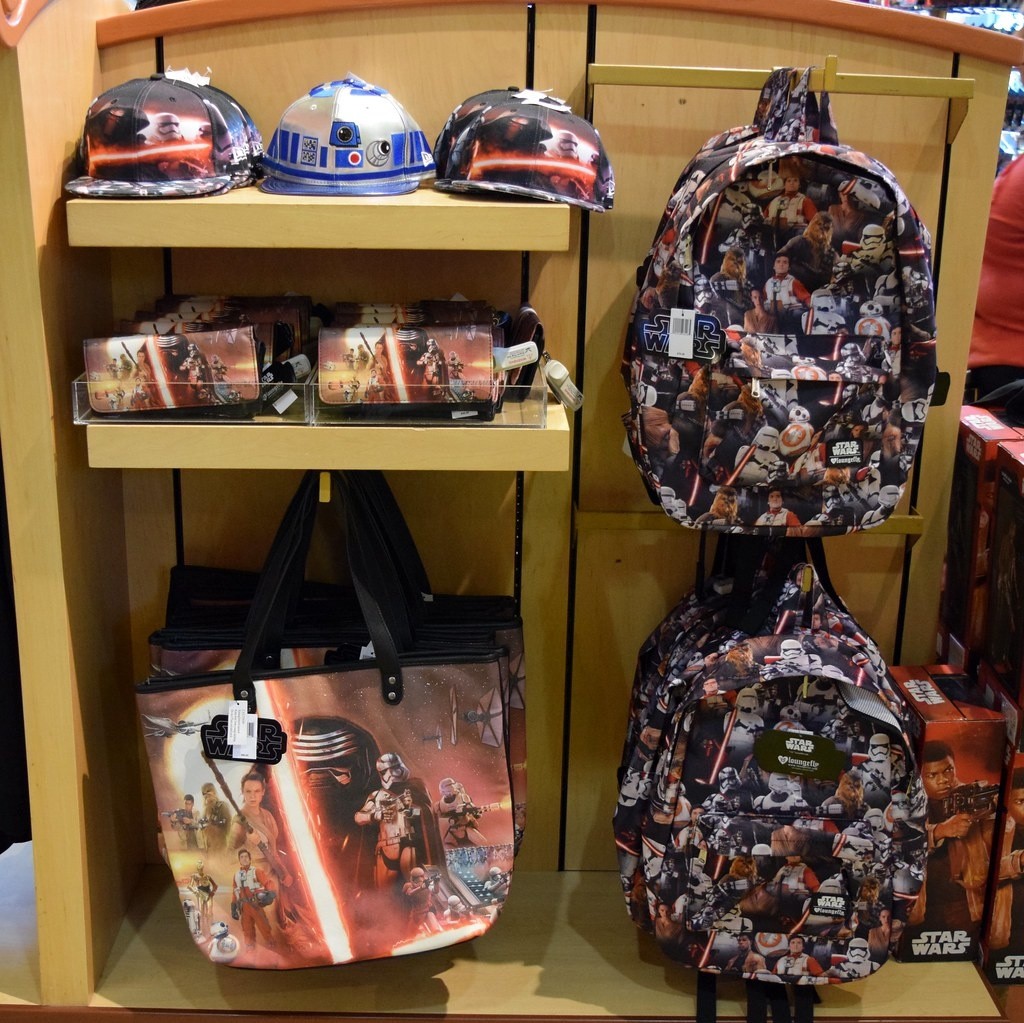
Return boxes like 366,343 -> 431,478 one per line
613,529 -> 930,987
621,68 -> 954,539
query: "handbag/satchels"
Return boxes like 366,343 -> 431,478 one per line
132,472 -> 529,972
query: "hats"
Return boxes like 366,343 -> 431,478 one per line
67,73 -> 617,214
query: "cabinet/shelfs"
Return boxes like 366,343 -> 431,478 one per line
66,187 -> 579,473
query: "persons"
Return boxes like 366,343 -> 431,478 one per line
967,154 -> 1024,417
903,741 -> 1023,982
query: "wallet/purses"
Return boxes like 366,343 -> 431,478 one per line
79,293 -> 559,416
